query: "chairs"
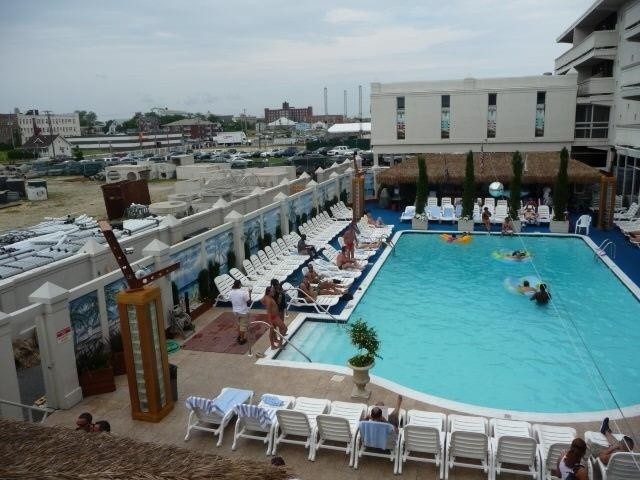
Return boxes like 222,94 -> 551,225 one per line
610,190 -> 640,251
534,424 -> 594,480
271,397 -> 333,459
311,401 -> 369,467
493,418 -> 542,479
183,389 -> 254,448
210,199 -> 395,318
398,409 -> 447,478
585,430 -> 640,480
167,304 -> 197,340
445,414 -> 493,478
231,394 -> 297,456
398,186 -> 594,237
353,406 -> 407,475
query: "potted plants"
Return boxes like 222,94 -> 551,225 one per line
506,147 -> 525,233
106,326 -> 127,376
456,147 -> 476,233
549,143 -> 573,234
411,149 -> 430,231
344,315 -> 382,401
77,339 -> 117,398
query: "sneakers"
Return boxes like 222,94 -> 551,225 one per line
256,351 -> 267,358
236,337 -> 248,345
600,417 -> 612,435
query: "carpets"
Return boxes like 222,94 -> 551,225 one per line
179,310 -> 270,356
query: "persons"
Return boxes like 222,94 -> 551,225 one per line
228,211 -> 387,350
365,394 -> 402,427
74,413 -> 92,433
93,421 -> 110,433
447,194 -> 552,305
556,417 -> 634,480
